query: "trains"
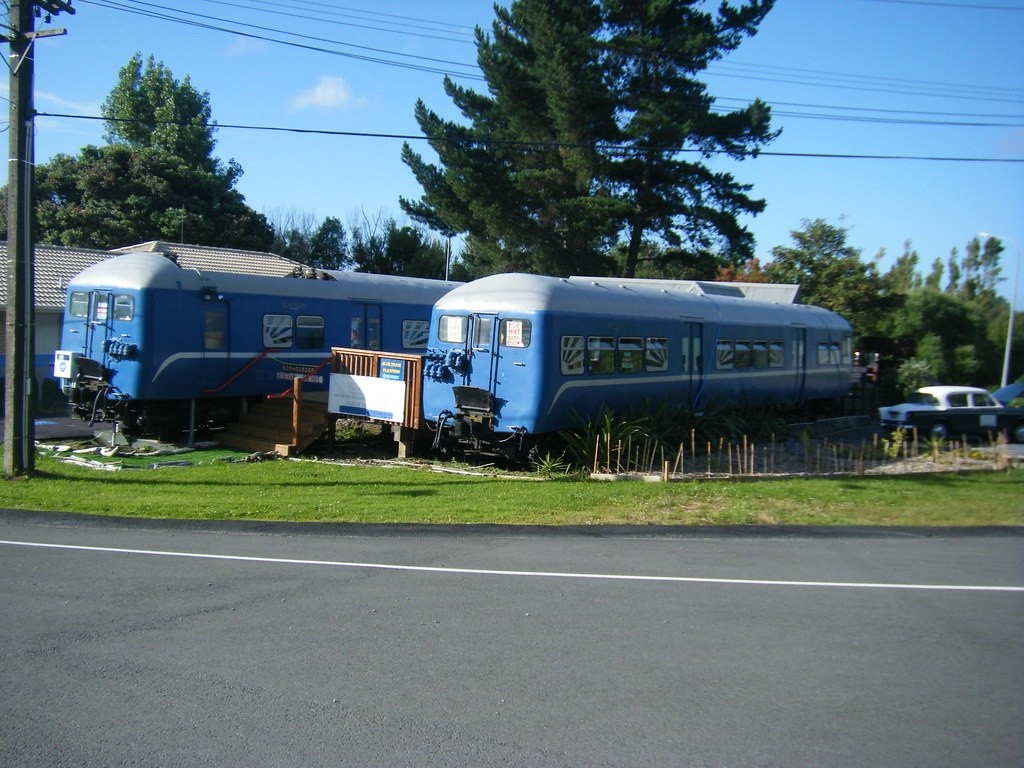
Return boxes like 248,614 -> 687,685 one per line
53,251 -> 465,442
420,272 -> 853,465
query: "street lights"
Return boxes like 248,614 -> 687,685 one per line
978,232 -> 1018,386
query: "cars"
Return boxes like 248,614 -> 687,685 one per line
878,377 -> 1024,443
